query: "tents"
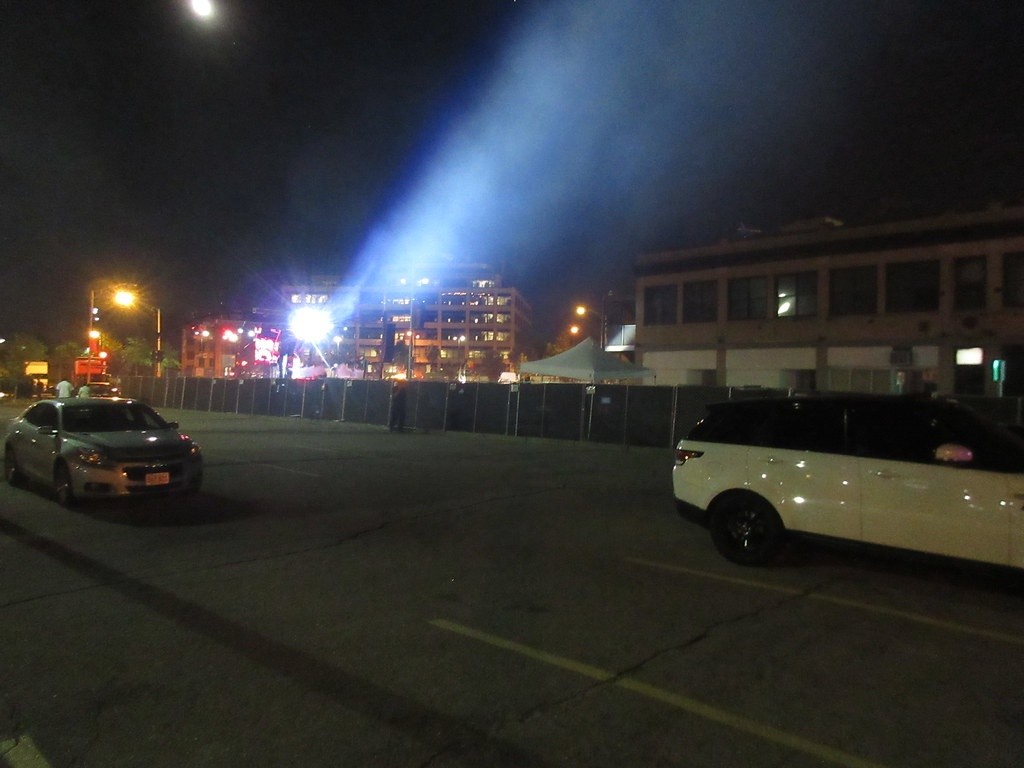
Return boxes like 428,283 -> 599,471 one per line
520,336 -> 656,383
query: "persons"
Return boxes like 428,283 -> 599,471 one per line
388,382 -> 408,433
56,376 -> 73,399
78,381 -> 90,399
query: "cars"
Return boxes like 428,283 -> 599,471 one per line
3,397 -> 201,508
670,390 -> 1024,610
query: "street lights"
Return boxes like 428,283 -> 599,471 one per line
115,292 -> 163,377
